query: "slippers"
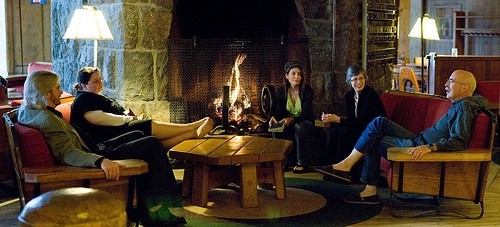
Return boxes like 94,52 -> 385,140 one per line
293,164 -> 310,173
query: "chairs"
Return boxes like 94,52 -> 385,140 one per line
399,67 -> 419,92
7,62 -> 53,95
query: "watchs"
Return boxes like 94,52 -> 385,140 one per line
428,143 -> 434,152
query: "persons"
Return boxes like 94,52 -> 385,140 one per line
70,66 -> 214,152
314,70 -> 489,203
17,71 -> 187,227
321,64 -> 388,165
269,62 -> 315,174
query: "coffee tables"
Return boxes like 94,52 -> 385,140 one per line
169,135 -> 293,208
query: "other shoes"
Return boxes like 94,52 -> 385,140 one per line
313,165 -> 351,182
139,205 -> 187,227
344,192 -> 381,204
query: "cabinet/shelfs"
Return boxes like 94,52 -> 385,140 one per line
435,5 -> 462,41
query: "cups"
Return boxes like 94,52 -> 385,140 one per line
323,114 -> 330,127
452,47 -> 457,57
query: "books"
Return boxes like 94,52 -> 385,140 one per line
268,123 -> 284,132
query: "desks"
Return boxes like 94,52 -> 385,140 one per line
393,68 -> 427,91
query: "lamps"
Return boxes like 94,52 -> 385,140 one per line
408,14 -> 441,94
63,5 -> 114,68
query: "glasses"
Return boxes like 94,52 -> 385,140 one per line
447,78 -> 466,85
350,77 -> 365,82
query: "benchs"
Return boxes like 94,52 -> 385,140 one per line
4,96 -> 147,227
378,90 -> 497,220
